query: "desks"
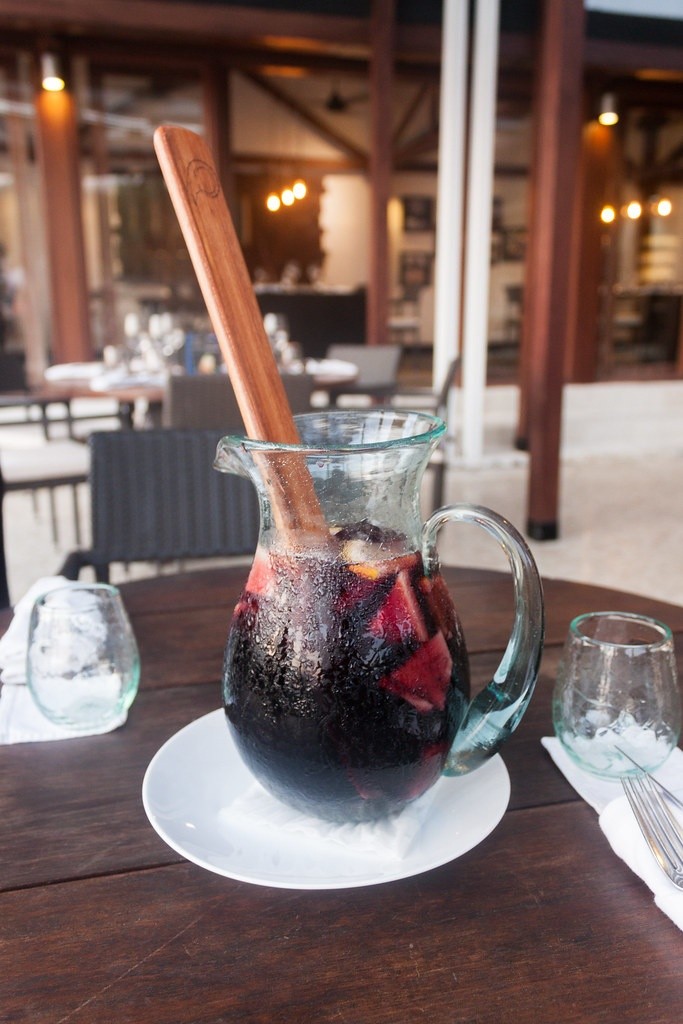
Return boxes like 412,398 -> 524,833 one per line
45,358 -> 358,423
0,561 -> 683,1024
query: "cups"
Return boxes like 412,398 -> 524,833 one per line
553,611 -> 681,781
27,584 -> 139,730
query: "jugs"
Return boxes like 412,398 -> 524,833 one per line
214,408 -> 544,821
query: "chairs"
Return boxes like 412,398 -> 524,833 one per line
0,341 -> 465,602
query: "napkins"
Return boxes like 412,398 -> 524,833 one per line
540,714 -> 683,931
0,576 -> 130,744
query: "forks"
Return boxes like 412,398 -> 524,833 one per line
622,775 -> 683,890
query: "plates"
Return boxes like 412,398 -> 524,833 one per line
141,708 -> 511,888
